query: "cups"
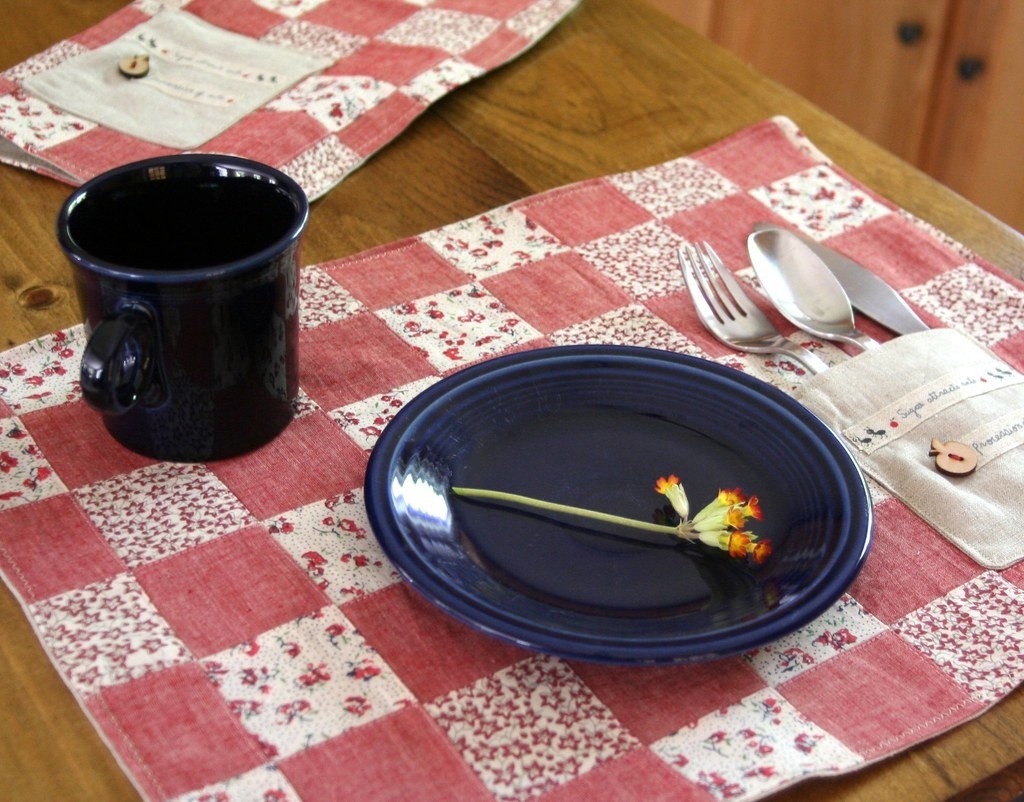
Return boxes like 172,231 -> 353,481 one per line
56,154 -> 308,461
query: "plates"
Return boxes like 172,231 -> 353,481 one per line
364,343 -> 873,663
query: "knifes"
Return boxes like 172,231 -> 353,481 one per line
754,222 -> 931,335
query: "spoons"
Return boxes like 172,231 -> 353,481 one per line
748,230 -> 880,352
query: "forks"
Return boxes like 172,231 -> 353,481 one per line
678,242 -> 829,376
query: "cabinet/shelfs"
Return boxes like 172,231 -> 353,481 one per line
651,0 -> 1024,233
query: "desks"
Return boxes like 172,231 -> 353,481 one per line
0,0 -> 1024,801
